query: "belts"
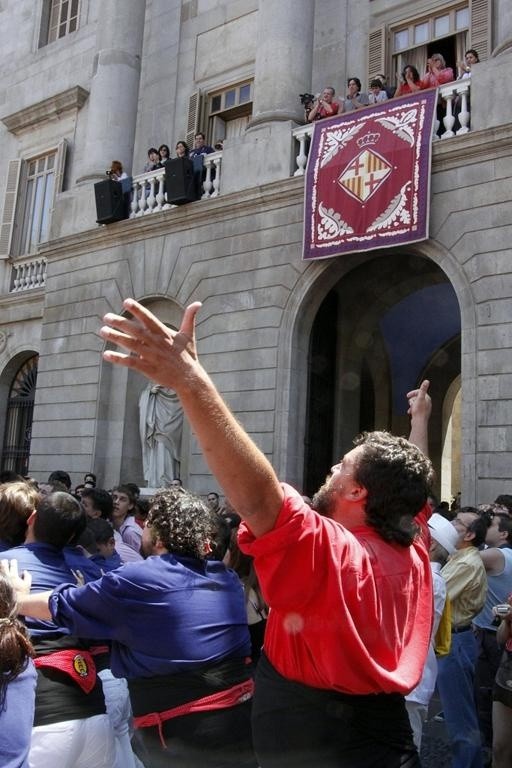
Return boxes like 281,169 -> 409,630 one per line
449,626 -> 474,633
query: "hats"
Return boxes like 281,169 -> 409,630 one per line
429,512 -> 458,556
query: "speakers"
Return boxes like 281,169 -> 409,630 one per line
164,158 -> 201,205
94,180 -> 125,224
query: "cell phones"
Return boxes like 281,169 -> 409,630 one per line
497,607 -> 508,614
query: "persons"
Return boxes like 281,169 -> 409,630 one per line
1,471 -> 511,767
306,49 -> 481,137
96,294 -> 435,766
140,132 -> 223,202
109,160 -> 128,181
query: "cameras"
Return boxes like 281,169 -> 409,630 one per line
106,170 -> 113,174
